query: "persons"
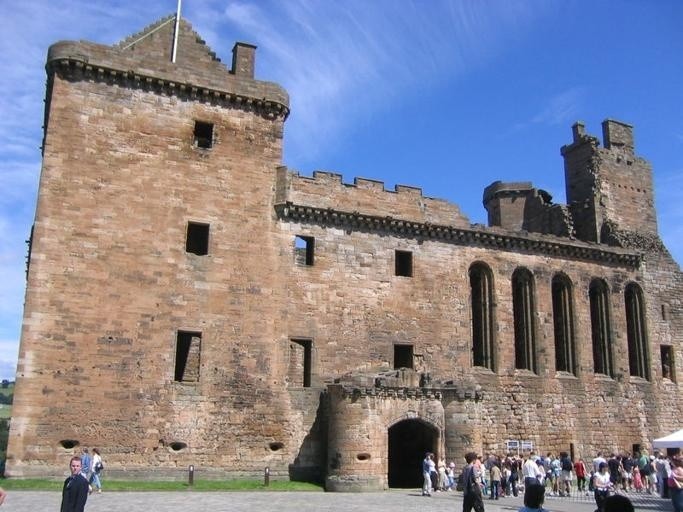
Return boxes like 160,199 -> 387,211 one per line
80,447 -> 92,495
59,456 -> 89,512
89,448 -> 102,494
0,487 -> 6,506
420,448 -> 682,512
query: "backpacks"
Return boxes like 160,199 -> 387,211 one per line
644,463 -> 652,476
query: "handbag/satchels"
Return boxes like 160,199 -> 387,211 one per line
467,466 -> 482,507
95,462 -> 104,473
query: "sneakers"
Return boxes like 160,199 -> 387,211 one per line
550,490 -> 571,497
